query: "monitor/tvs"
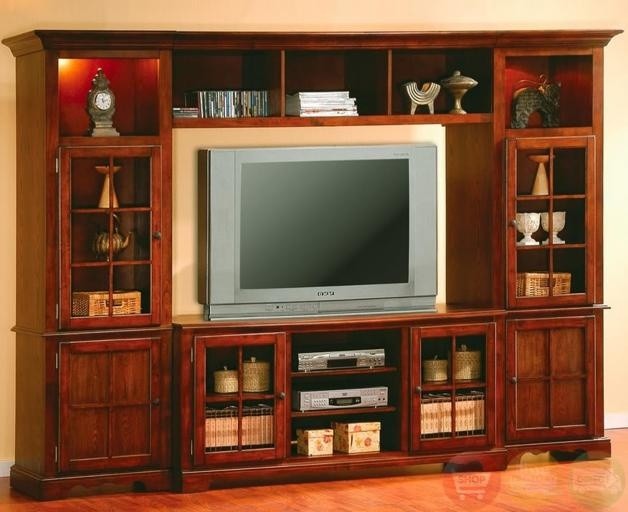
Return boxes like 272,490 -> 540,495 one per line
197,141 -> 439,322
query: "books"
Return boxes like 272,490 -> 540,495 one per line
204,401 -> 275,455
172,87 -> 359,119
420,389 -> 485,442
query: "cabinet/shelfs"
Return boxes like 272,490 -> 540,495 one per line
0,29 -> 623,501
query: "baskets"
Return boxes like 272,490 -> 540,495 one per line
516,272 -> 570,297
72,291 -> 141,318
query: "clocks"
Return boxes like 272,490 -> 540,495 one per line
85,66 -> 121,137
